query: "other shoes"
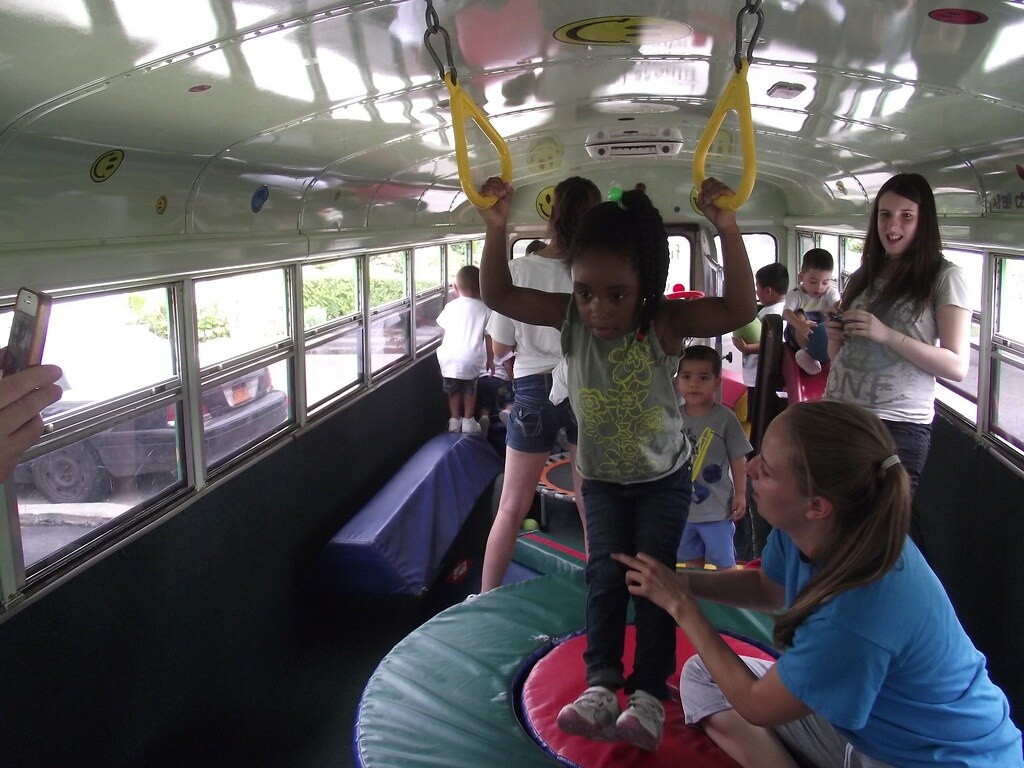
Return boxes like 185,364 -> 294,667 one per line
478,415 -> 490,438
499,409 -> 511,425
795,350 -> 820,375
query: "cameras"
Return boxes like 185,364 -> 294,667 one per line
828,311 -> 852,324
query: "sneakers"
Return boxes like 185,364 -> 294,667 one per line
616,689 -> 665,751
462,417 -> 481,433
557,686 -> 622,742
448,417 -> 463,432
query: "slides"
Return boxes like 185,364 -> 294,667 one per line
781,342 -> 832,406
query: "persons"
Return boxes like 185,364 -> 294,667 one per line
0,348 -> 62,481
676,345 -> 754,569
479,177 -> 757,751
822,173 -> 973,506
611,400 -> 1024,768
782,249 -> 842,375
465,177 -> 602,602
732,263 -> 789,423
436,240 -> 550,434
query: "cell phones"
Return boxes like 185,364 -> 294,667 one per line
1,286 -> 54,378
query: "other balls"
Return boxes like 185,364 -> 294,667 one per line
522,518 -> 538,531
732,315 -> 762,344
